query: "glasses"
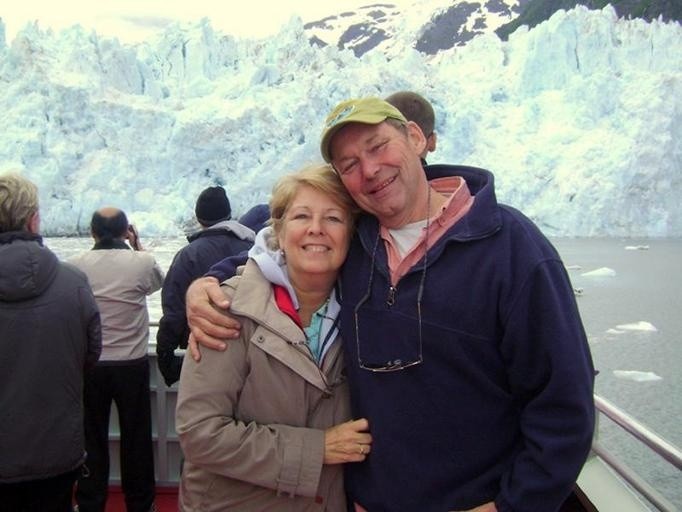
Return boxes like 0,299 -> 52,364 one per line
352,301 -> 426,377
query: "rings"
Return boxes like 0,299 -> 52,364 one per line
359,443 -> 364,455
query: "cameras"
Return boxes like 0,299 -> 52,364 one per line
127,226 -> 132,237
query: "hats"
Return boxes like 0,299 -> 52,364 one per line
194,186 -> 233,225
320,95 -> 408,164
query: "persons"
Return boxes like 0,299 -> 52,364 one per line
154,185 -> 259,388
0,171 -> 103,512
382,90 -> 437,166
185,94 -> 598,510
174,163 -> 372,510
64,206 -> 167,510
